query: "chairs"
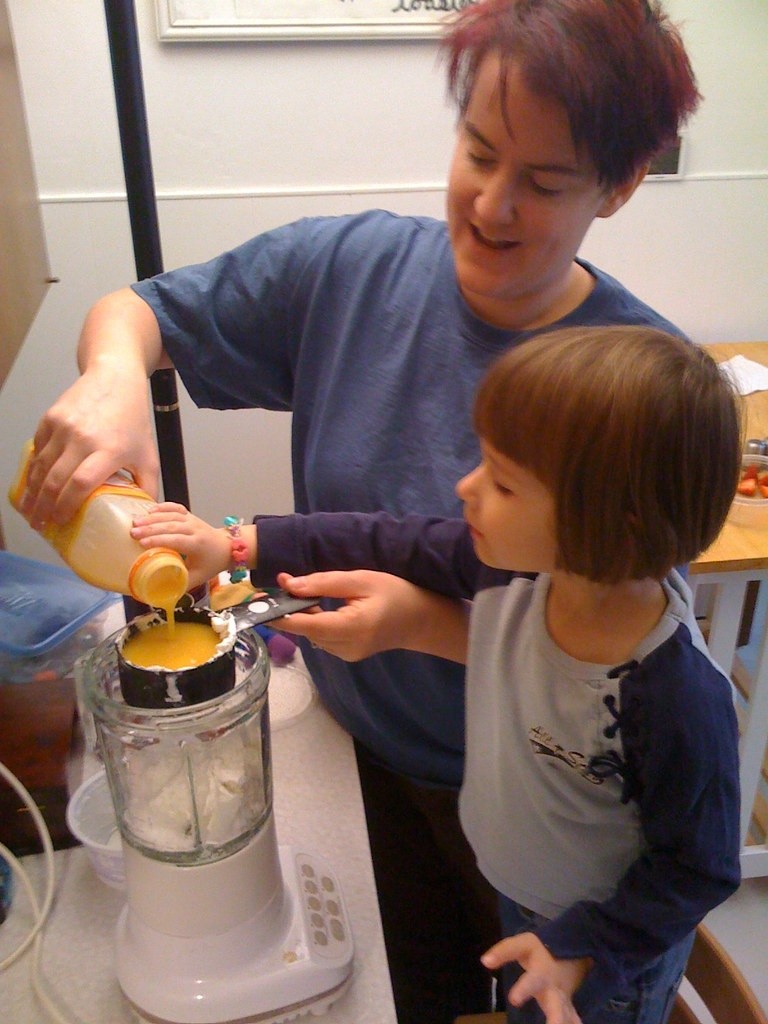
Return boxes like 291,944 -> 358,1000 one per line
455,922 -> 768,1024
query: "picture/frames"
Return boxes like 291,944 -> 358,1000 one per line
155,0 -> 486,40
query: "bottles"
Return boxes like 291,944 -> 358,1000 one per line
6,436 -> 189,608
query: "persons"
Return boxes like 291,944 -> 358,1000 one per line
20,0 -> 705,1023
130,328 -> 741,1024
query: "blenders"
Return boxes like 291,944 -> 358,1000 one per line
79,628 -> 358,1024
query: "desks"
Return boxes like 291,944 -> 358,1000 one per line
685,340 -> 768,879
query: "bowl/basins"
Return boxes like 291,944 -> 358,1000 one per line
726,452 -> 768,526
64,769 -> 125,891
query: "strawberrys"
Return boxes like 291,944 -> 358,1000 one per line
736,464 -> 768,500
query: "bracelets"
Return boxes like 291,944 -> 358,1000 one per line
224,515 -> 252,588
